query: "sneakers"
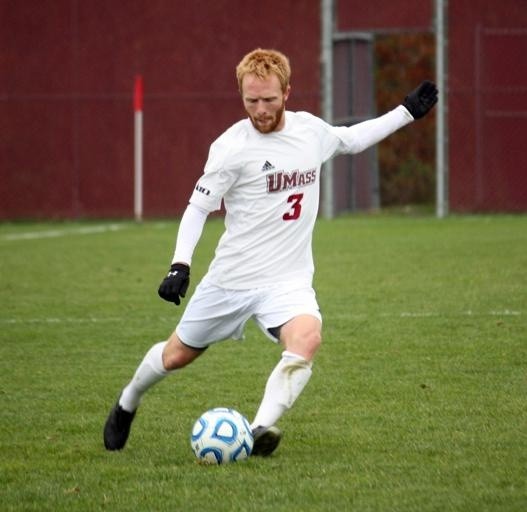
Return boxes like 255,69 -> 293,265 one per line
251,425 -> 281,456
103,393 -> 137,451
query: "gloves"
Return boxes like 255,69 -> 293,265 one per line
158,264 -> 190,305
401,80 -> 439,121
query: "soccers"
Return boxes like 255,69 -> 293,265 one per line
191,408 -> 254,463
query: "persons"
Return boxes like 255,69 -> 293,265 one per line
103,46 -> 440,474
